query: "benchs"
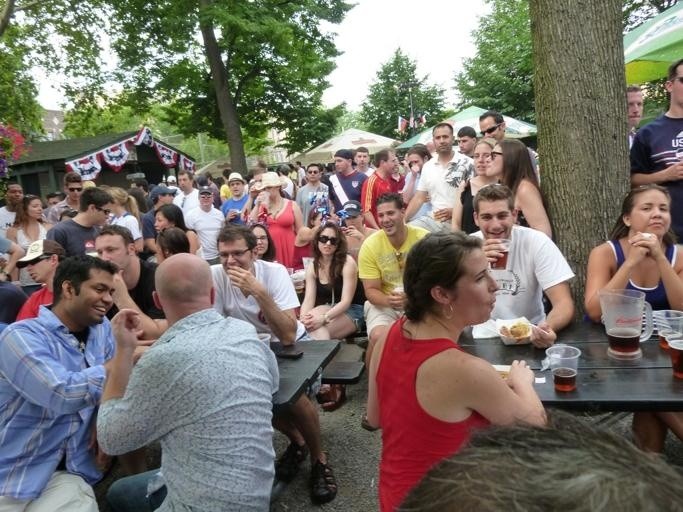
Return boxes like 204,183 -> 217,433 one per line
343,330 -> 368,344
318,342 -> 366,385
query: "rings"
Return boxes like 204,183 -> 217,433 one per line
641,231 -> 652,238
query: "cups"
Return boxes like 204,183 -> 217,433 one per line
665,335 -> 683,380
545,345 -> 582,391
259,191 -> 271,208
259,332 -> 272,345
655,309 -> 683,350
490,238 -> 513,269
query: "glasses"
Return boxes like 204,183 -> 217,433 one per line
201,196 -> 212,198
217,248 -> 249,257
23,256 -> 51,267
308,170 -> 319,174
480,123 -> 501,136
490,151 -> 503,159
96,207 -> 112,214
255,236 -> 268,240
68,187 -> 82,191
318,236 -> 339,245
159,228 -> 167,259
164,193 -> 176,197
395,251 -> 405,272
670,76 -> 683,83
23,194 -> 39,199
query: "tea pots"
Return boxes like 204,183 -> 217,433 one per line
597,286 -> 654,361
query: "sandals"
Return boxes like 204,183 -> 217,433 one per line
321,383 -> 346,412
316,383 -> 337,404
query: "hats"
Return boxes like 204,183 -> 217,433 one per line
343,200 -> 362,216
228,172 -> 246,186
254,172 -> 288,189
15,240 -> 65,268
199,186 -> 212,194
151,186 -> 177,199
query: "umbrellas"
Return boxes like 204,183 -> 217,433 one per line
623,0 -> 683,84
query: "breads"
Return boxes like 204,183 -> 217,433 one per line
500,322 -> 529,338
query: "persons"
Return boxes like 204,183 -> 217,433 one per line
0,106 -> 576,512
396,409 -> 683,512
627,86 -> 643,149
581,183 -> 683,454
630,59 -> 683,244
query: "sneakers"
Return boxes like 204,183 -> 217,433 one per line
274,442 -> 309,484
310,451 -> 338,505
362,412 -> 378,431
